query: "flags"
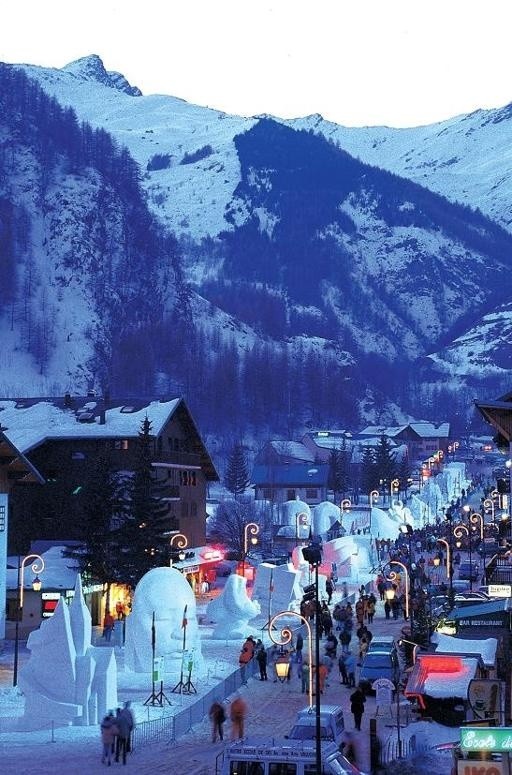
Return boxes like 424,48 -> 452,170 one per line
181,605 -> 189,629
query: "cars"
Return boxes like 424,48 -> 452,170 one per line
370,637 -> 396,647
356,648 -> 400,692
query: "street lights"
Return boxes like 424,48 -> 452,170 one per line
268,610 -> 321,774
339,499 -> 352,536
169,534 -> 188,568
243,522 -> 260,576
381,489 -> 502,641
13,553 -> 46,686
295,512 -> 311,546
369,441 -> 460,532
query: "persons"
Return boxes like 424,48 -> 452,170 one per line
349,686 -> 367,731
229,694 -> 246,742
131,567 -> 199,675
101,700 -> 136,767
103,611 -> 113,642
209,697 -> 226,743
238,470 -> 498,696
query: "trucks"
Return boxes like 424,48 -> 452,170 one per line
216,736 -> 367,775
285,704 -> 346,744
